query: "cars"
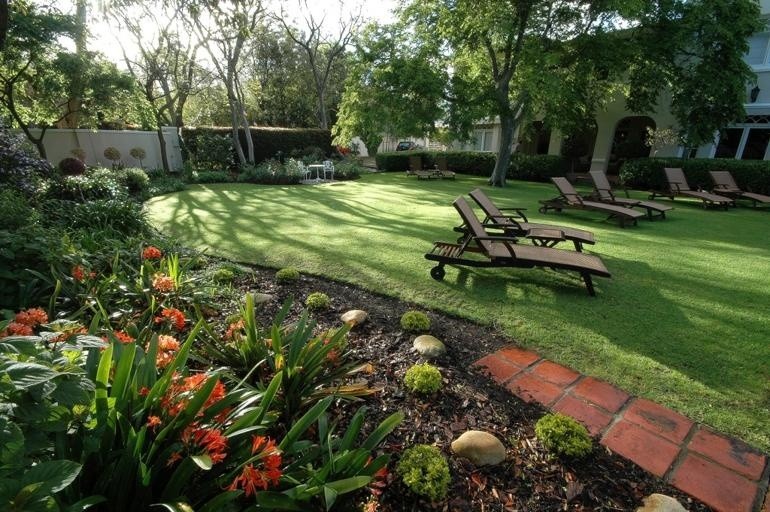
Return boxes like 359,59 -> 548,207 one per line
396,141 -> 425,150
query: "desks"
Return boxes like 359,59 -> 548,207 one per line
309,164 -> 325,183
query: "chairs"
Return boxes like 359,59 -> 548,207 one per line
581,170 -> 673,222
709,171 -> 770,209
296,160 -> 312,180
323,160 -> 335,181
424,195 -> 611,296
452,187 -> 596,254
648,168 -> 734,212
433,157 -> 456,180
538,176 -> 646,229
407,155 -> 434,180
